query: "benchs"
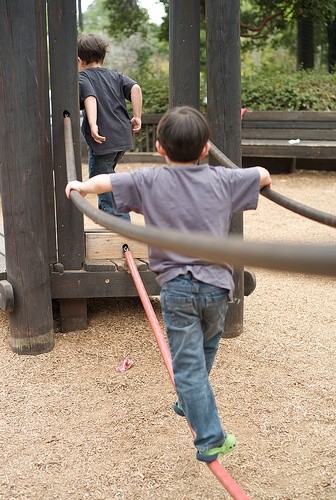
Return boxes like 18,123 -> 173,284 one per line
241,110 -> 336,173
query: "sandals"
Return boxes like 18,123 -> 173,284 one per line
173,401 -> 185,417
196,434 -> 237,462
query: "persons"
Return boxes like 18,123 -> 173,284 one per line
65,107 -> 271,462
77,33 -> 143,223
